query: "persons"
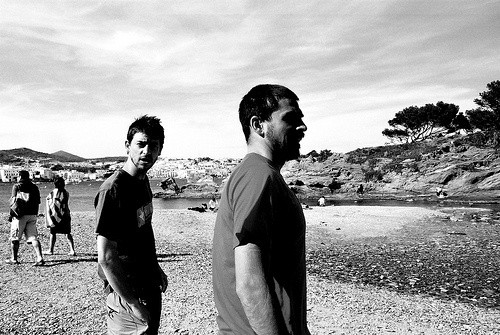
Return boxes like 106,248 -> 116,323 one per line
44,177 -> 76,255
208,198 -> 219,211
94,114 -> 168,335
4,170 -> 45,267
212,83 -> 312,335
318,196 -> 326,206
